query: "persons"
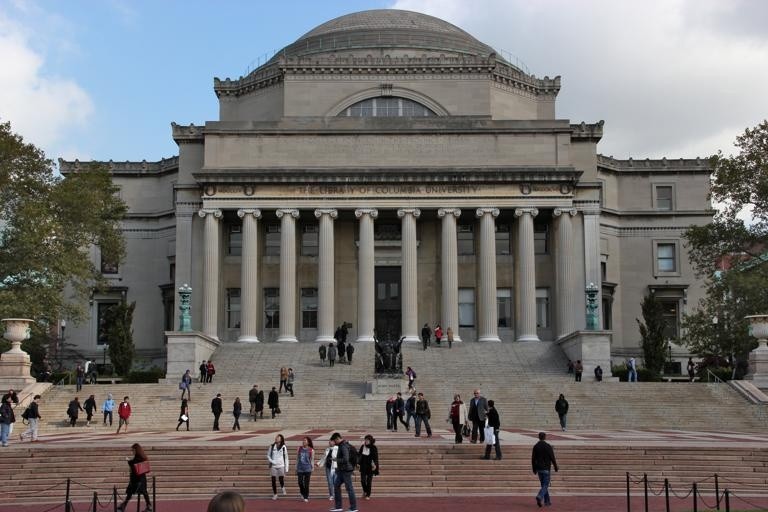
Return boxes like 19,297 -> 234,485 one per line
9,388 -> 19,403
319,320 -> 354,367
67,358 -> 133,435
627,355 -> 638,382
205,490 -> 247,512
20,394 -> 43,442
264,430 -> 385,512
595,365 -> 602,381
0,394 -> 15,447
687,357 -> 697,383
385,366 -> 503,461
575,360 -> 583,381
566,360 -> 574,373
116,442 -> 152,511
421,322 -> 453,351
177,358 -> 294,431
555,393 -> 570,431
530,430 -> 559,507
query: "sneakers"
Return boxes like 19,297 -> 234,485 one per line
272,487 -> 370,512
536,494 -> 543,508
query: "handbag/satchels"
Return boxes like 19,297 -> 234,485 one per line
484,426 -> 496,445
181,414 -> 189,422
275,407 -> 282,415
462,425 -> 471,437
21,408 -> 29,421
133,461 -> 152,475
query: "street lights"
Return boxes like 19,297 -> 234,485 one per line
103,343 -> 107,365
583,282 -> 602,330
711,316 -> 719,370
668,341 -> 672,377
58,319 -> 66,369
178,284 -> 193,333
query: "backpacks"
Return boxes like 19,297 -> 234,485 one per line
343,443 -> 358,466
